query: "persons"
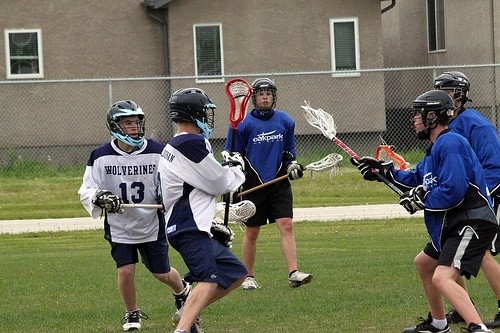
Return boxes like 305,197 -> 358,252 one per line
156,85 -> 247,333
430,70 -> 500,330
76,98 -> 193,332
223,75 -> 315,291
350,89 -> 494,333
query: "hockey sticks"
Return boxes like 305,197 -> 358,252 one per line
374,146 -> 412,170
223,79 -> 254,227
301,105 -> 405,196
105,200 -> 257,223
237,153 -> 344,198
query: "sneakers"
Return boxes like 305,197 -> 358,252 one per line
288,271 -> 313,288
467,322 -> 495,333
401,311 -> 452,333
242,276 -> 261,290
173,279 -> 193,312
121,308 -> 149,331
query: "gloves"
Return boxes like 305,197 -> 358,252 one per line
92,190 -> 124,214
285,160 -> 306,180
399,185 -> 430,214
350,155 -> 394,182
221,150 -> 246,171
211,221 -> 235,249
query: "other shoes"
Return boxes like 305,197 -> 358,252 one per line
172,308 -> 204,333
445,297 -> 478,325
483,299 -> 500,329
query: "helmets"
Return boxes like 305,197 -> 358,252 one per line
251,78 -> 278,116
106,99 -> 146,147
411,90 -> 455,141
169,87 -> 217,140
434,71 -> 472,112
